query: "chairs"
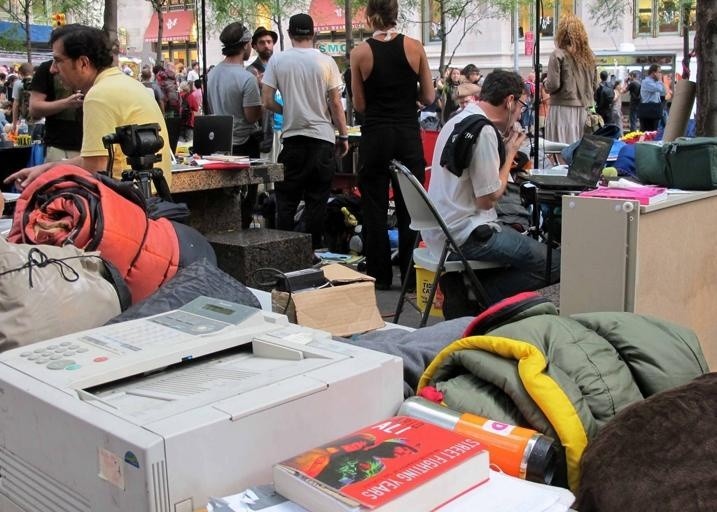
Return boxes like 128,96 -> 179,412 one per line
391,158 -> 513,329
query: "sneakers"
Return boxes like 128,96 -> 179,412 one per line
440,271 -> 469,320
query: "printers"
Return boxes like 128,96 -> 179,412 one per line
0,297 -> 404,512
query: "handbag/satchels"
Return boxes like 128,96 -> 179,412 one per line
635,137 -> 717,190
599,84 -> 614,110
638,102 -> 663,120
585,113 -> 604,134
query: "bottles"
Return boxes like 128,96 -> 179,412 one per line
18,119 -> 29,142
394,397 -> 565,487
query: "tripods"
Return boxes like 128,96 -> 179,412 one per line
121,170 -> 174,202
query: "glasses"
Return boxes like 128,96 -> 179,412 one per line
517,99 -> 528,113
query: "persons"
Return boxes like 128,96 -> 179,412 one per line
1,1 -> 699,322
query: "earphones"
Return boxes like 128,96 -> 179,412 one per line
509,102 -> 512,105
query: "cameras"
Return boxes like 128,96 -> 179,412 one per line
104,122 -> 164,169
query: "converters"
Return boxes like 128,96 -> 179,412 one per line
276,268 -> 324,291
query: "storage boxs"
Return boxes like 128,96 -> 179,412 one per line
271,262 -> 386,338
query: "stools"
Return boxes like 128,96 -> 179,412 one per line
206,228 -> 313,288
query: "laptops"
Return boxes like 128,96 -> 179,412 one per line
520,133 -> 614,189
194,115 -> 234,158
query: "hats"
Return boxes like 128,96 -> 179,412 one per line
220,23 -> 252,46
289,14 -> 313,35
251,27 -> 277,46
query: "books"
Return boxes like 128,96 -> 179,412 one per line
271,416 -> 490,512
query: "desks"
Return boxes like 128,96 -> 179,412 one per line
171,158 -> 284,236
0,139 -> 48,193
